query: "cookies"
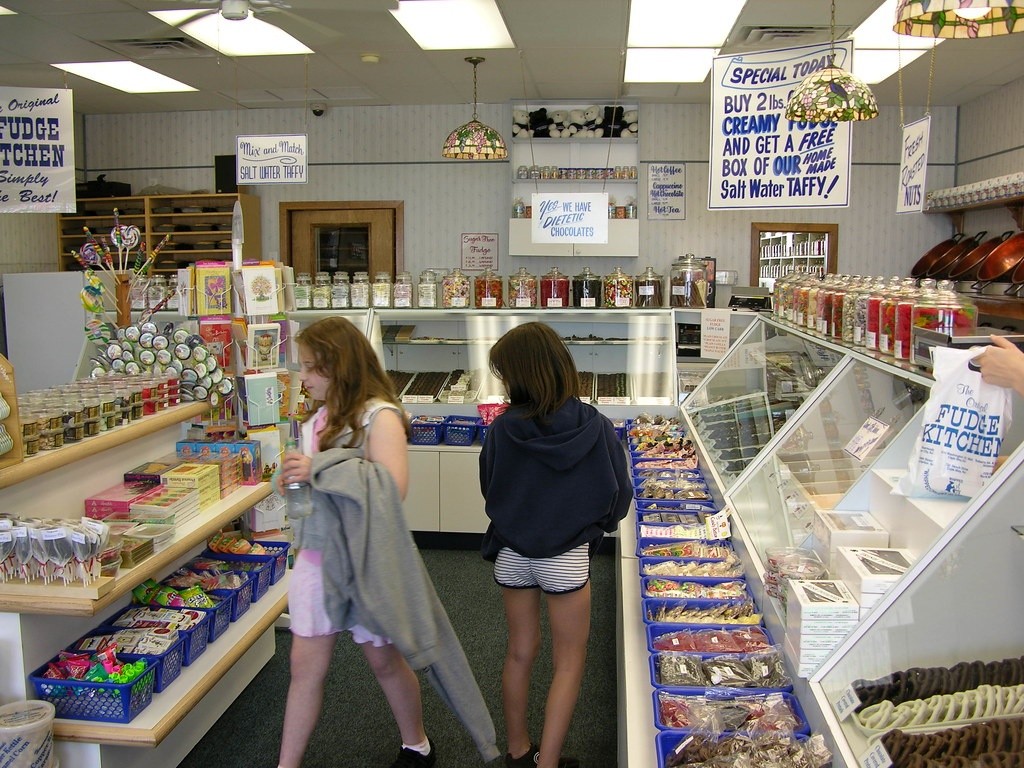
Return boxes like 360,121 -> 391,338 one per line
852,657 -> 1024,768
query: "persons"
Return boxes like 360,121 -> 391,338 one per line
478,323 -> 635,768
974,334 -> 1024,397
276,317 -> 436,768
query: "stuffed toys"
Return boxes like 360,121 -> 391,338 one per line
585,105 -> 604,138
512,110 -> 534,138
621,110 -> 638,137
600,106 -> 628,137
529,108 -> 553,137
565,110 -> 588,138
547,110 -> 571,138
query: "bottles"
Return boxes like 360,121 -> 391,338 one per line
772,271 -> 978,367
759,235 -> 826,277
294,269 -> 437,309
129,274 -> 181,311
283,438 -> 313,518
441,253 -> 706,309
614,166 -> 638,179
518,165 -> 558,179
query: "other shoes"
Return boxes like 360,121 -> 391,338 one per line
390,735 -> 435,768
504,744 -> 580,768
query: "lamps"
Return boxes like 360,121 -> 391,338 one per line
785,0 -> 879,123
892,0 -> 1024,39
442,56 -> 507,160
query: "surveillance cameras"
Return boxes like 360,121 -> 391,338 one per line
310,104 -> 325,116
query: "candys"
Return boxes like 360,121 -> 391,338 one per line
71,207 -> 177,347
0,515 -> 109,586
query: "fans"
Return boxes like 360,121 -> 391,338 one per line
131,0 -> 398,40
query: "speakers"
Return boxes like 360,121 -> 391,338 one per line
214,155 -> 239,194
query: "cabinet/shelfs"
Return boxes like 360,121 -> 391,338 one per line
58,193 -> 262,277
922,195 -> 1024,319
615,313 -> 1024,768
507,99 -> 642,257
0,395 -> 293,768
293,305 -> 770,553
761,233 -> 828,280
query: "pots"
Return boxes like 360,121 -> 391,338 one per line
971,231 -> 1024,295
911,232 -> 966,279
924,231 -> 988,279
948,231 -> 1016,279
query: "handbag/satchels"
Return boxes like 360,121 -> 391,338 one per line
889,346 -> 1012,501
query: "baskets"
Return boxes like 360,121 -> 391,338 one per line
27,652 -> 160,724
63,625 -> 188,693
201,540 -> 292,585
609,418 -> 626,441
162,567 -> 258,621
476,417 -> 491,446
626,418 -> 832,768
128,583 -> 237,643
186,555 -> 275,604
442,415 -> 481,445
410,415 -> 448,445
97,599 -> 215,667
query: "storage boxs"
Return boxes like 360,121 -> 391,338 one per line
85,452 -> 244,527
176,439 -> 263,485
773,448 -> 882,514
381,325 -> 417,344
784,510 -> 917,677
